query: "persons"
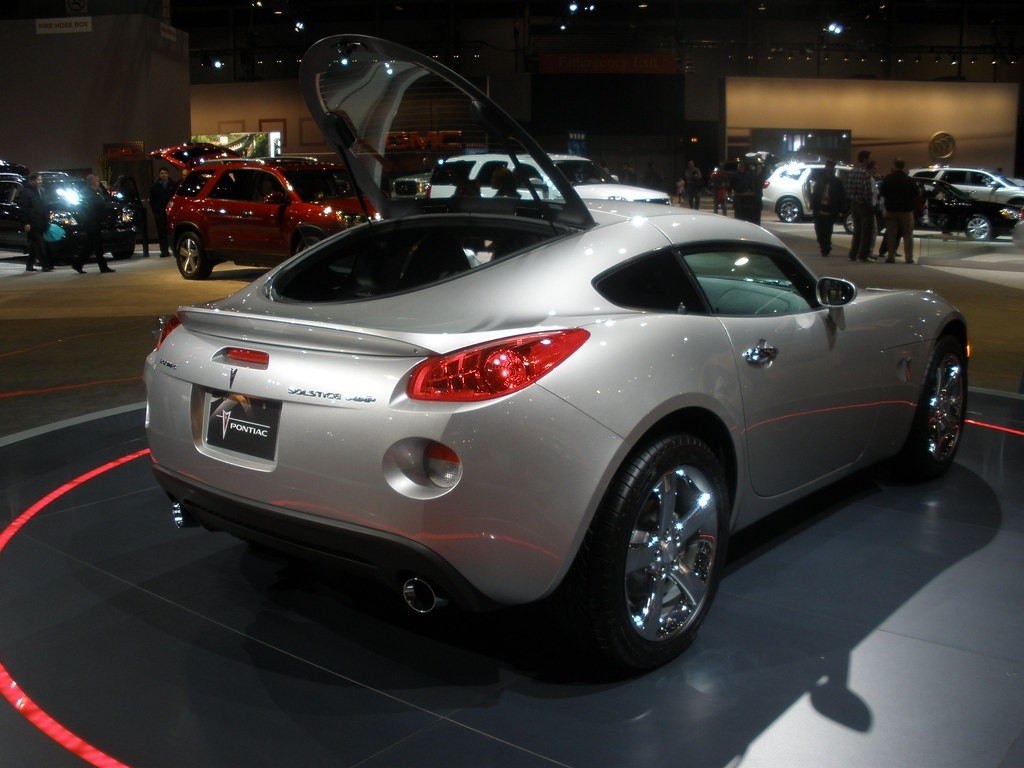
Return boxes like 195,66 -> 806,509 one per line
810,150 -> 926,264
602,160 -> 766,226
448,160 -> 532,263
20,167 -> 178,274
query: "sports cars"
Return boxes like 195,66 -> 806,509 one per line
139,33 -> 971,669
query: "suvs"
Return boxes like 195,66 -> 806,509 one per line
907,168 -> 1024,207
149,142 -> 384,279
425,154 -> 671,204
0,170 -> 147,265
762,164 -> 856,224
711,157 -> 778,208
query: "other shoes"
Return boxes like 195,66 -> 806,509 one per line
879,253 -> 884,257
848,257 -> 856,261
906,261 -> 914,263
160,252 -> 169,257
887,259 -> 896,263
822,252 -> 828,256
857,257 -> 875,262
43,269 -> 51,271
32,269 -> 37,271
896,254 -> 901,256
73,266 -> 86,273
102,269 -> 115,273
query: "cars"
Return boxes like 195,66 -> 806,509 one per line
982,177 -> 1024,187
775,152 -> 847,168
391,168 -> 435,199
1013,213 -> 1024,249
842,177 -> 1022,241
